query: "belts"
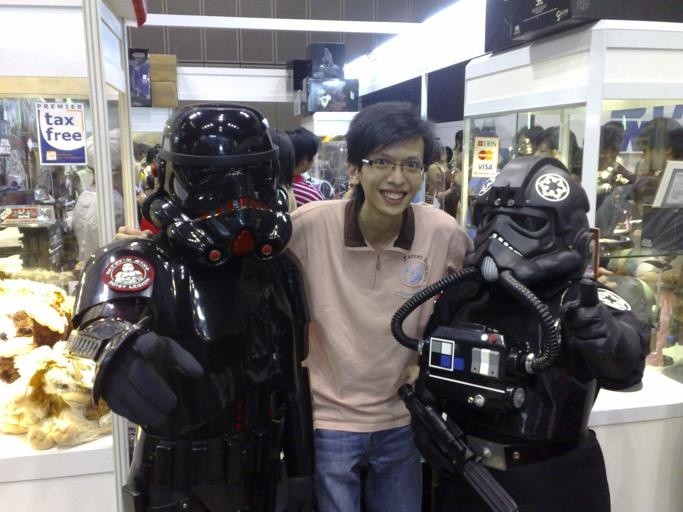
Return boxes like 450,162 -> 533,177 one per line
466,430 -> 592,472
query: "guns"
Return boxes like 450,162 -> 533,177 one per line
398,382 -> 518,511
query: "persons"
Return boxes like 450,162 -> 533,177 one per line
385,152 -> 649,512
276,99 -> 477,510
269,129 -> 299,212
288,126 -> 324,209
77,102 -> 324,512
71,128 -> 125,268
426,113 -> 682,258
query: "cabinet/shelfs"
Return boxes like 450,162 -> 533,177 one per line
422,98 -> 682,382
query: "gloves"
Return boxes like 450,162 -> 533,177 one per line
560,279 -> 620,362
99,329 -> 203,428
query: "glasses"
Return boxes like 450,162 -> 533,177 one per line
360,155 -> 427,177
